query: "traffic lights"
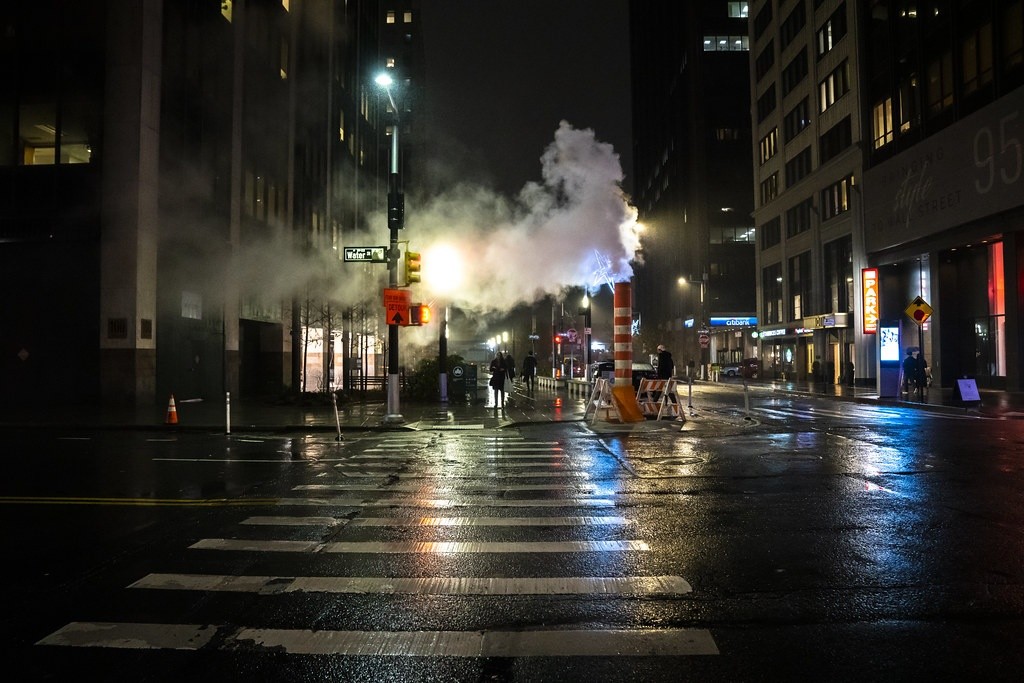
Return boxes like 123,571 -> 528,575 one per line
405,251 -> 421,285
411,304 -> 431,324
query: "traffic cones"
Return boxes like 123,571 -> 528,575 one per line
164,392 -> 179,425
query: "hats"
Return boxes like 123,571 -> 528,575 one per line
658,345 -> 665,351
907,352 -> 912,355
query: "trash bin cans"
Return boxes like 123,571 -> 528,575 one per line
448,364 -> 478,403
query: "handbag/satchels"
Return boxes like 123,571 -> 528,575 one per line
504,371 -> 514,392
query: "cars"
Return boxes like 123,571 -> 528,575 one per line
710,357 -> 759,380
560,353 -> 659,398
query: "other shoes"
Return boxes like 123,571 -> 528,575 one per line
902,391 -> 909,394
913,388 -> 918,393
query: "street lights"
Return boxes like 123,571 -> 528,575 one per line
374,71 -> 403,424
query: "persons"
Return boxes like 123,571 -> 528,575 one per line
654,345 -> 676,403
689,358 -> 695,377
902,352 -> 927,394
812,358 -> 821,383
523,351 -> 537,391
490,352 -> 507,407
505,355 -> 515,381
